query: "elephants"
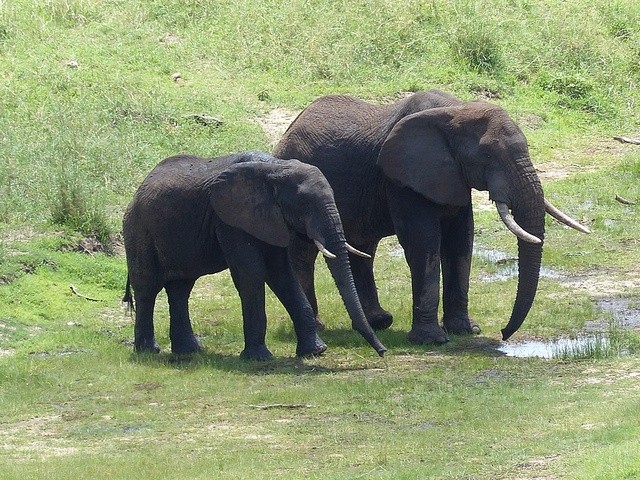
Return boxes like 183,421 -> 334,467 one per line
123,150 -> 388,360
272,88 -> 590,347
